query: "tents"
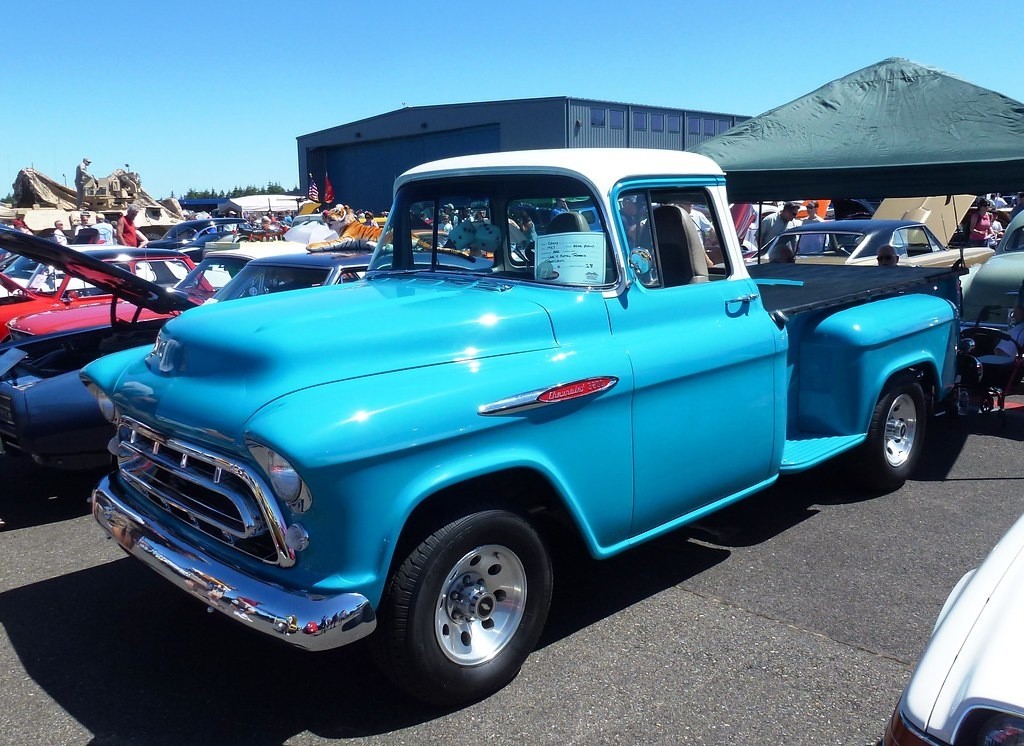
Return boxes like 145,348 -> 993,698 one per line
218,195 -> 320,219
636,57 -> 1024,256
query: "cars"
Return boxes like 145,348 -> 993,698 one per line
682,194 -> 1024,273
954,207 -> 1024,393
0,225 -> 493,504
869,510 -> 1024,745
0,203 -> 627,318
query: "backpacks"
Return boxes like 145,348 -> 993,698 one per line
963,212 -> 992,244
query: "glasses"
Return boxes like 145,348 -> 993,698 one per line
981,204 -> 990,207
790,209 -> 796,216
877,256 -> 895,261
806,205 -> 815,209
365,217 -> 371,219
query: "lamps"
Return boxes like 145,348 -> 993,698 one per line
576,120 -> 583,127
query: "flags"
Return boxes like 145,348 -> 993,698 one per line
309,179 -> 319,201
324,177 -> 334,203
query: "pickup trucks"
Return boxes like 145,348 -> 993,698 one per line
80,142 -> 970,702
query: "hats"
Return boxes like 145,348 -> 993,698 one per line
284,210 -> 291,212
444,203 -> 454,210
229,211 -> 236,214
83,158 -> 92,163
261,216 -> 271,223
96,213 -> 105,219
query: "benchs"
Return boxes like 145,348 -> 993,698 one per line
845,245 -> 932,257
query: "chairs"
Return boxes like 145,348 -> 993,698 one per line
638,205 -> 710,286
546,211 -> 590,234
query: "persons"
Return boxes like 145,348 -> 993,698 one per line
680,203 -> 715,248
75,158 -> 93,210
754,202 -> 799,254
351,209 -> 389,228
0,205 -> 148,289
223,211 -> 237,238
245,210 -> 297,241
551,198 -> 569,220
420,213 -> 425,221
797,201 -> 829,253
187,215 -> 216,235
969,193 -> 1024,248
619,196 -> 713,266
438,204 -> 458,247
519,211 -> 536,242
464,208 -> 488,222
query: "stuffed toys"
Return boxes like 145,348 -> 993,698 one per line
306,204 -> 393,252
